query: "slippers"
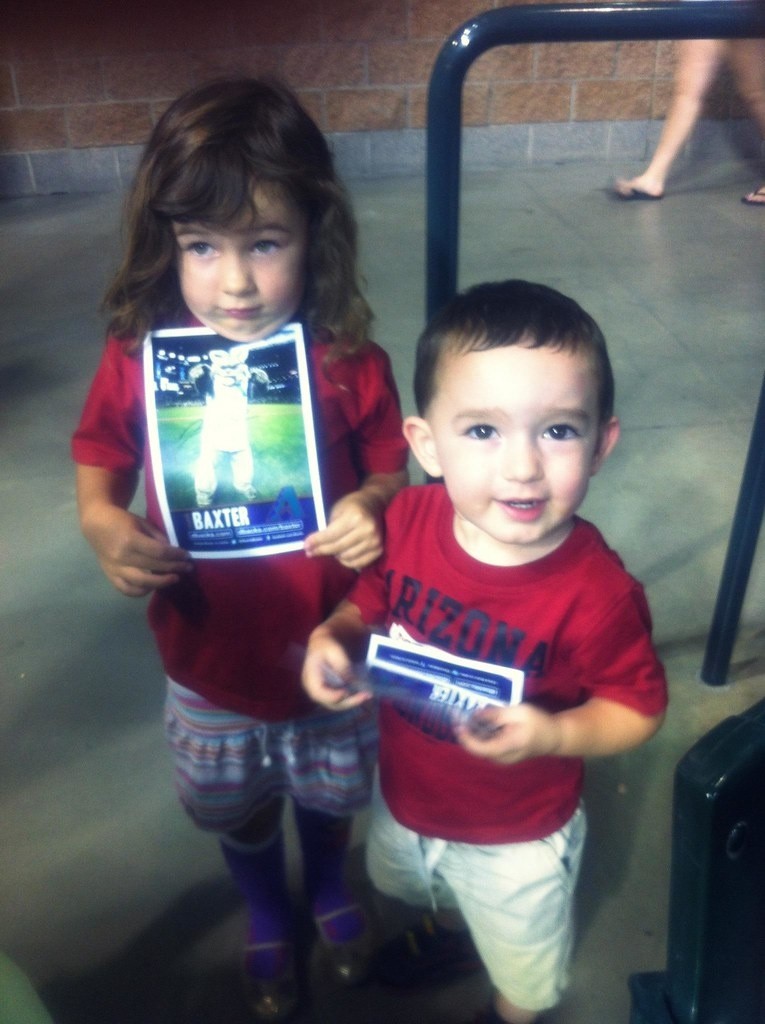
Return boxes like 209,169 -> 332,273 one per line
741,186 -> 765,206
612,179 -> 665,201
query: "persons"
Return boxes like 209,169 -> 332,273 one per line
727,38 -> 765,205
299,276 -> 671,1024
612,37 -> 725,205
70,72 -> 409,984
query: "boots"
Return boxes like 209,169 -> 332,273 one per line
295,820 -> 369,949
220,822 -> 301,983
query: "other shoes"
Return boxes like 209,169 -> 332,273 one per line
370,911 -> 472,990
